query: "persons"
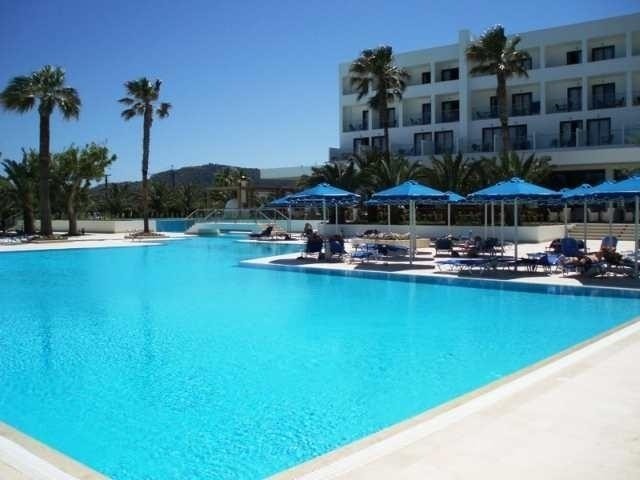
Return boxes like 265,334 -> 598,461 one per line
249,224 -> 272,237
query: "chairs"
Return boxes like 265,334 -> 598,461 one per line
248,223 -> 639,277
